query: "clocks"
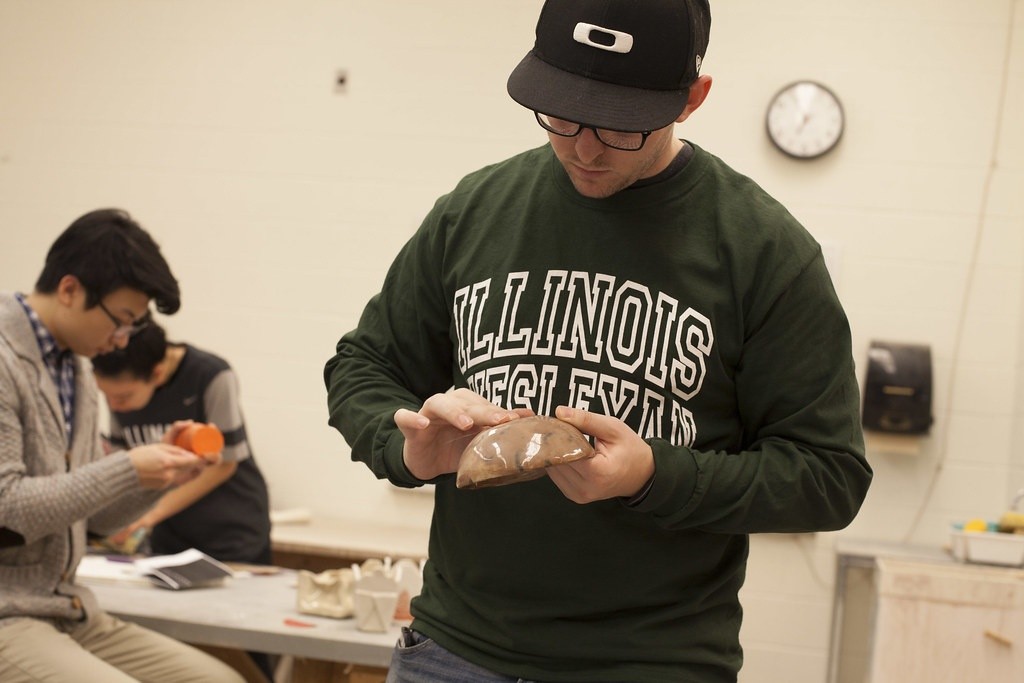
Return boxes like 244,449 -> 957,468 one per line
765,80 -> 847,161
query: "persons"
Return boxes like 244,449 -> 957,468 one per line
90,309 -> 273,565
0,207 -> 246,683
323,0 -> 874,683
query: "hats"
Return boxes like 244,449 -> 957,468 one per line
507,0 -> 711,132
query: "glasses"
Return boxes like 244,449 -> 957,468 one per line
533,108 -> 652,151
97,299 -> 151,337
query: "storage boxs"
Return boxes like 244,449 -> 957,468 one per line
953,529 -> 1024,566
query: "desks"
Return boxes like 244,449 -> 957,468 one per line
74,548 -> 412,668
272,510 -> 434,562
